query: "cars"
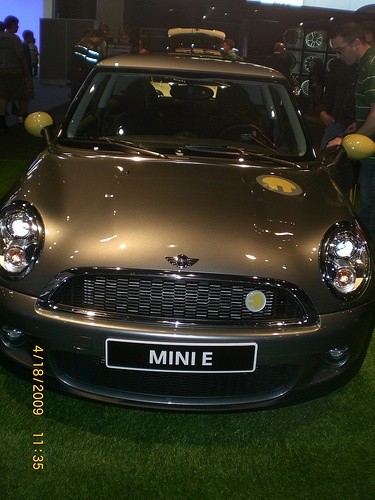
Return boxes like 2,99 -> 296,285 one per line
0,52 -> 375,415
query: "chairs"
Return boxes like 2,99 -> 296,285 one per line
216,85 -> 251,114
123,80 -> 164,134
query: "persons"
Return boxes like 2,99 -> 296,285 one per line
260,20 -> 375,250
198,86 -> 270,148
221,39 -> 238,60
0,15 -> 129,132
